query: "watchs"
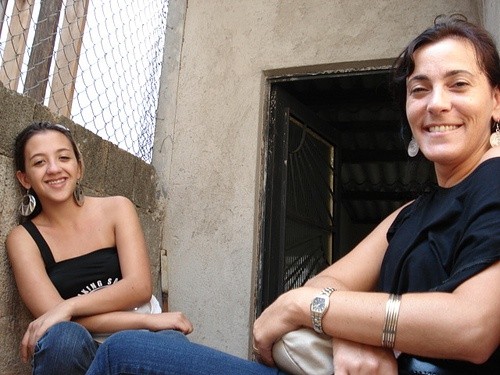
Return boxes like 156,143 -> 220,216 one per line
310,287 -> 335,339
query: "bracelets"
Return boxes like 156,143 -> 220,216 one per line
382,293 -> 402,350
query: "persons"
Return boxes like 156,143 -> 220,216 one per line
87,14 -> 500,375
6,121 -> 194,375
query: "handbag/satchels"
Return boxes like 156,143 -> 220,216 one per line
271,328 -> 334,375
87,286 -> 162,344
